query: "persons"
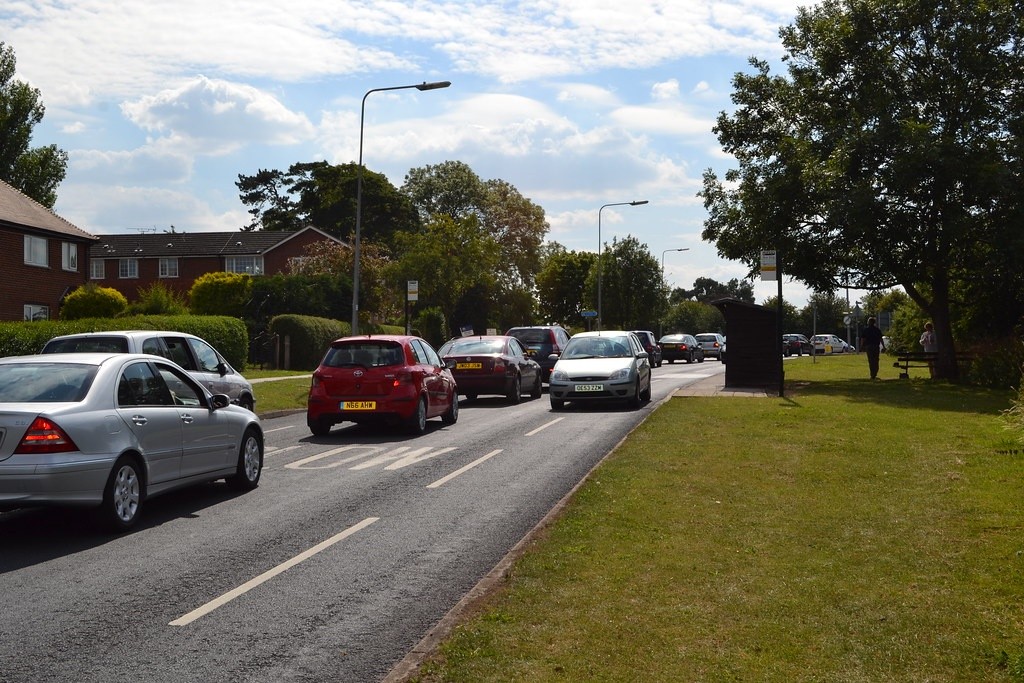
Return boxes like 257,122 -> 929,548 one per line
858,317 -> 887,382
919,322 -> 939,381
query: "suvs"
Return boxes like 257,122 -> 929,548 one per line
783,333 -> 814,358
632,330 -> 662,368
695,333 -> 726,362
508,326 -> 572,385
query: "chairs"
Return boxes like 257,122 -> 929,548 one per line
5,348 -> 399,401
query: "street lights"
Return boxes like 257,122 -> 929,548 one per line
855,300 -> 864,354
348,81 -> 451,335
660,248 -> 689,283
597,200 -> 649,331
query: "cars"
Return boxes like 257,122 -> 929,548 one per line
549,331 -> 652,409
810,334 -> 846,354
658,334 -> 705,364
309,334 -> 460,436
39,330 -> 255,417
0,353 -> 267,532
839,339 -> 855,353
435,336 -> 542,407
